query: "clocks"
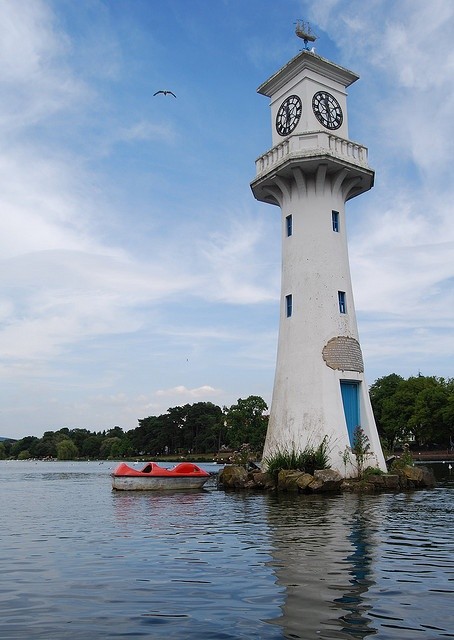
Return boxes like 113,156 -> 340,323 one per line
311,91 -> 343,130
275,95 -> 302,138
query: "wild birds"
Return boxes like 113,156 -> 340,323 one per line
153,90 -> 177,98
310,47 -> 317,53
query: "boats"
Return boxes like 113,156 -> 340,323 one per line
111,462 -> 209,491
